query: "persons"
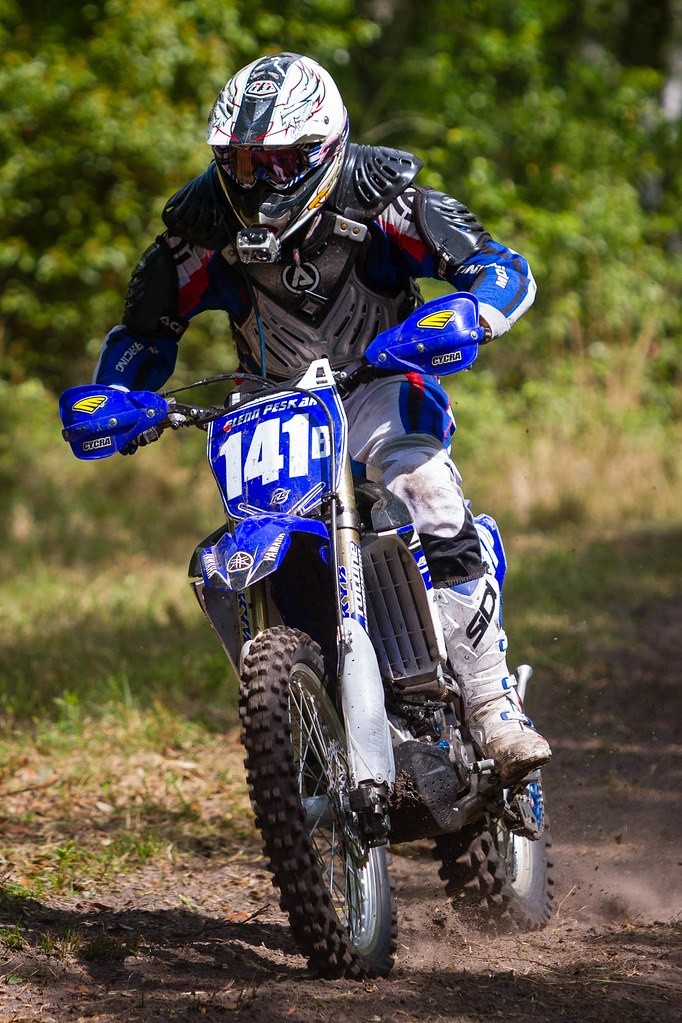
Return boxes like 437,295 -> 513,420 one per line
93,51 -> 553,782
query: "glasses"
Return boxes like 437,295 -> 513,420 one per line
212,144 -> 315,191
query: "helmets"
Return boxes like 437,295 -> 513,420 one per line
204,52 -> 350,243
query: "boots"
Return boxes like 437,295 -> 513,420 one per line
432,574 -> 553,789
